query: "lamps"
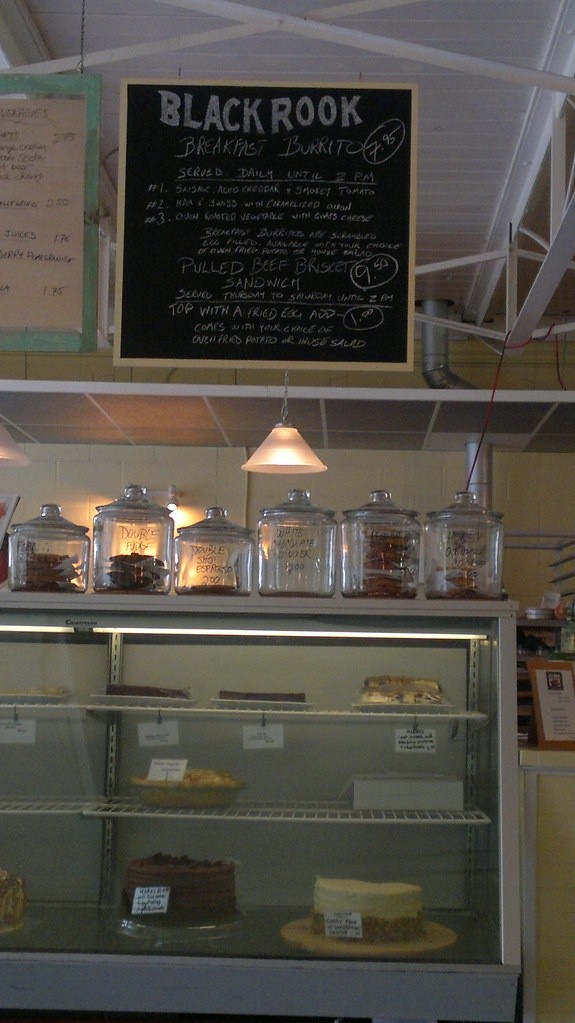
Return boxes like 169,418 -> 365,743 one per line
242,369 -> 328,475
1,625 -> 489,640
165,485 -> 180,510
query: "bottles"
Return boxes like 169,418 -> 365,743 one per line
421,491 -> 505,599
93,484 -> 174,595
173,508 -> 254,595
340,491 -> 421,600
7,503 -> 91,592
258,490 -> 337,598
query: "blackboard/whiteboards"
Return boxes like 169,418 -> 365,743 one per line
111,78 -> 417,372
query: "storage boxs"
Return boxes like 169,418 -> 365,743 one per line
340,773 -> 467,811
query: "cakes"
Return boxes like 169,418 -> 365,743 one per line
121,852 -> 236,925
313,878 -> 424,944
0,869 -> 24,932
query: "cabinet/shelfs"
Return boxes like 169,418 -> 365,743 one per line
517,618 -> 575,738
0,639 -> 523,1022
